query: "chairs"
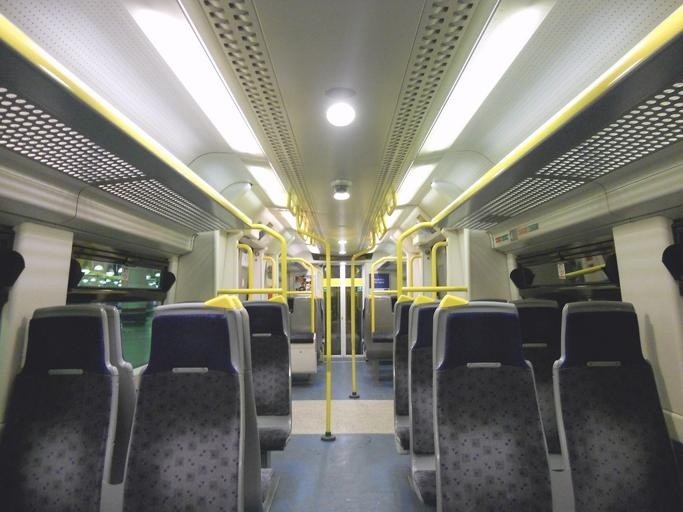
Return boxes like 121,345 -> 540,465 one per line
431,303 -> 555,511
118,302 -> 246,511
0,305 -> 120,511
551,298 -> 683,512
362,297 -> 398,383
97,301 -> 295,481
391,298 -> 561,509
287,294 -> 327,386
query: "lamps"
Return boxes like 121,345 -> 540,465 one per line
331,179 -> 352,200
